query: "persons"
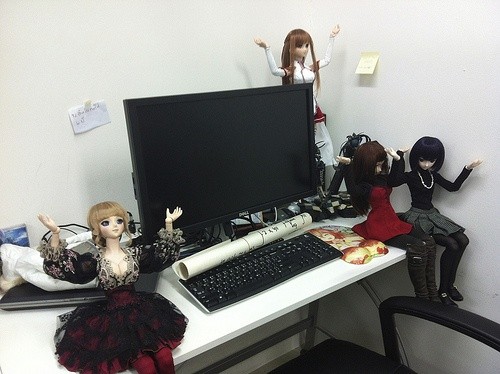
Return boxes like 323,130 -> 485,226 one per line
390,136 -> 484,308
36,201 -> 189,374
336,140 -> 441,304
253,23 -> 342,201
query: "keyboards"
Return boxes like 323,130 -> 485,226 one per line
177,231 -> 344,313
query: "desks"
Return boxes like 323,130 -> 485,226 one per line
0,212 -> 412,374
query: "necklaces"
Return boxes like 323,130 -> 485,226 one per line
418,171 -> 434,189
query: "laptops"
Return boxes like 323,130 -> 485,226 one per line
0,254 -> 163,310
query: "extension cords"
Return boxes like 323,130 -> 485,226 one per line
119,229 -> 141,249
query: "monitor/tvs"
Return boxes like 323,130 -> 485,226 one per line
123,83 -> 317,260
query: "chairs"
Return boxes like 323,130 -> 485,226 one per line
263,296 -> 500,374
